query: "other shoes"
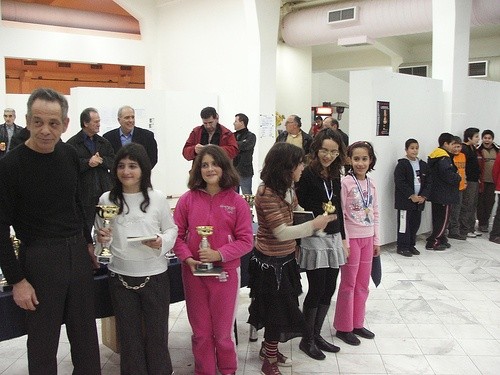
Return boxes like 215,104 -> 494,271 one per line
397,248 -> 412,257
447,233 -> 466,240
426,242 -> 447,250
442,240 -> 451,248
466,232 -> 476,237
474,232 -> 483,236
335,330 -> 360,345
489,235 -> 500,244
410,247 -> 420,255
353,327 -> 375,339
479,224 -> 489,232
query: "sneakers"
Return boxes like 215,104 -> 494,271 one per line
259,341 -> 293,367
260,359 -> 282,375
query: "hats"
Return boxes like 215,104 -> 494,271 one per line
314,115 -> 322,120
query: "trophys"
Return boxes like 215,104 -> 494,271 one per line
315,202 -> 335,237
95,204 -> 120,263
195,225 -> 213,271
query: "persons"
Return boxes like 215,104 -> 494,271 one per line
393,138 -> 432,256
0,87 -> 348,375
425,127 -> 500,250
332,141 -> 379,347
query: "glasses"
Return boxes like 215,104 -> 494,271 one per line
319,149 -> 339,158
285,120 -> 295,124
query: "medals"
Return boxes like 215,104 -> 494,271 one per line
365,207 -> 370,213
328,201 -> 333,205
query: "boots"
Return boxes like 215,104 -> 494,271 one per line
314,304 -> 340,352
299,303 -> 326,360
